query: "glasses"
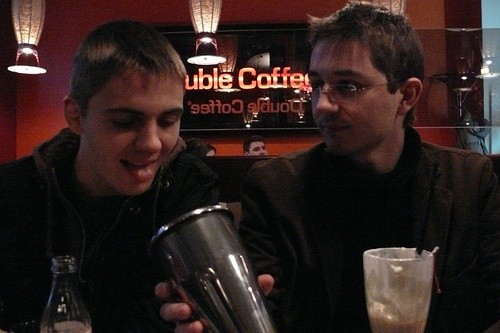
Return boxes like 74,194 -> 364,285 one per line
299,77 -> 405,103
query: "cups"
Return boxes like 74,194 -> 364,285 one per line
363,248 -> 434,333
157,204 -> 276,333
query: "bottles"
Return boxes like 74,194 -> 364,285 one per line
40,256 -> 92,333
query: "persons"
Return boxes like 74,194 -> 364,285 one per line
154,1 -> 500,333
0,19 -> 219,333
187,135 -> 268,156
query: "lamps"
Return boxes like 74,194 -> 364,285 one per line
186,0 -> 227,66
7,0 -> 47,74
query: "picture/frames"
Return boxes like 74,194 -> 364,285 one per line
158,21 -> 321,136
